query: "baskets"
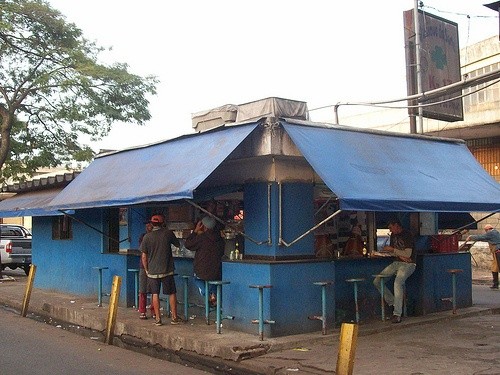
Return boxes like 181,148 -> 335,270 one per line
429,234 -> 459,253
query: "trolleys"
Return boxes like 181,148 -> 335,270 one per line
458,238 -> 479,268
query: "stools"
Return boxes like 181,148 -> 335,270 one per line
447,269 -> 464,314
308,281 -> 333,335
128,269 -> 139,308
208,281 -> 234,334
248,285 -> 275,341
345,278 -> 366,325
93,267 -> 110,307
372,275 -> 391,321
395,273 -> 407,317
167,274 -> 178,318
196,277 -> 216,324
178,275 -> 197,320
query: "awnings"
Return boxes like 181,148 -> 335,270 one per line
0,183 -> 75,218
438,212 -> 477,229
280,122 -> 500,212
48,122 -> 260,211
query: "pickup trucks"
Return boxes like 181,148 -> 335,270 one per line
0,223 -> 33,276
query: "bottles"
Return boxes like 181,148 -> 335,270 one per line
362,241 -> 368,257
176,246 -> 181,255
234,241 -> 239,260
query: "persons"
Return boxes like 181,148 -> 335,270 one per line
184,216 -> 225,306
139,220 -> 156,320
466,224 -> 500,290
139,215 -> 186,326
373,218 -> 417,323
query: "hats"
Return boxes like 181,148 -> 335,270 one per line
144,216 -> 165,224
202,216 -> 216,229
484,225 -> 492,231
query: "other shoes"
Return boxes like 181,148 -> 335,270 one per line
171,316 -> 187,325
208,294 -> 216,312
391,316 -> 400,323
140,314 -> 146,319
490,283 -> 499,288
152,322 -> 162,326
384,305 -> 394,310
151,313 -> 156,319
